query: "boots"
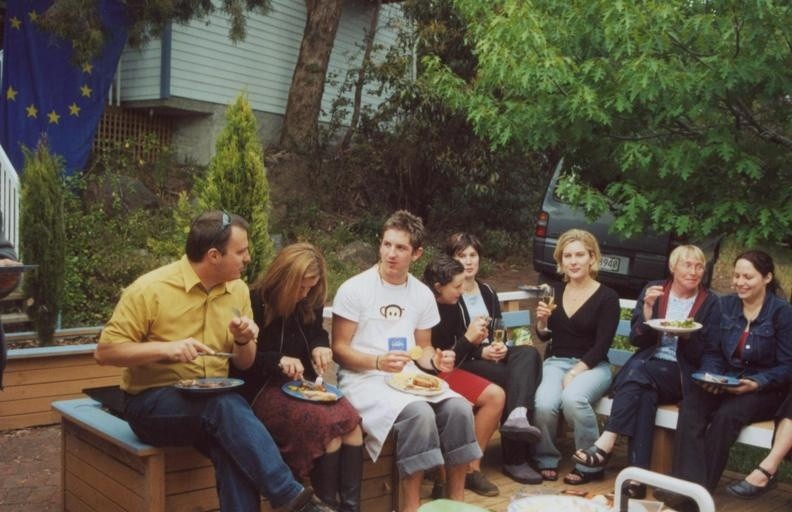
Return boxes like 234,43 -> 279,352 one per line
312,447 -> 344,512
340,444 -> 363,512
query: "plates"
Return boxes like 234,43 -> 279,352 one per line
174,377 -> 245,395
0,263 -> 41,274
690,372 -> 742,387
646,318 -> 705,334
281,379 -> 344,402
384,371 -> 450,396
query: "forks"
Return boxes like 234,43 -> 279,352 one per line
277,361 -> 316,389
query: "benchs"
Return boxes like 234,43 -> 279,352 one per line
52,290 -> 535,512
535,286 -> 792,489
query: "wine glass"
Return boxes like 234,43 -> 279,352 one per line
535,287 -> 556,334
490,320 -> 506,367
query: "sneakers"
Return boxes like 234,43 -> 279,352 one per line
467,470 -> 498,497
502,461 -> 544,484
432,479 -> 443,501
501,417 -> 541,442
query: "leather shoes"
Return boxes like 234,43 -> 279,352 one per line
727,465 -> 779,500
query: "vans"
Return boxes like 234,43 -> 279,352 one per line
532,148 -> 721,294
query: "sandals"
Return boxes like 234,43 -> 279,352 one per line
606,483 -> 646,501
574,443 -> 612,465
566,467 -> 605,484
543,464 -> 558,482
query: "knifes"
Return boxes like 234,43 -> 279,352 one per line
315,371 -> 322,385
195,350 -> 237,359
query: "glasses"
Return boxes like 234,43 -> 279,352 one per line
683,266 -> 705,273
206,205 -> 231,243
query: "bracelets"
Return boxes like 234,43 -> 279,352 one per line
234,339 -> 255,347
375,354 -> 383,371
430,359 -> 441,376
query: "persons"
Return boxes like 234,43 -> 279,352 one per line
725,392 -> 792,501
331,210 -> 484,512
93,210 -> 336,512
533,229 -> 614,486
570,245 -> 720,499
652,250 -> 792,511
229,243 -> 364,512
445,232 -> 544,485
422,256 -> 506,500
